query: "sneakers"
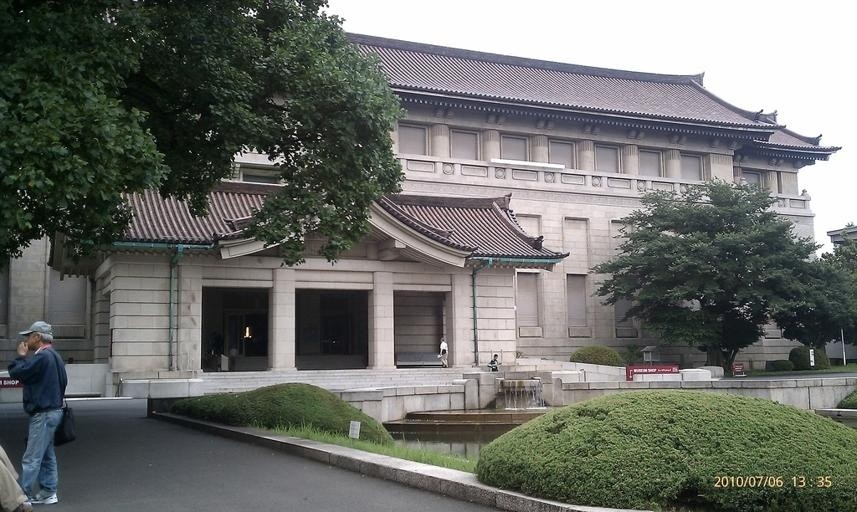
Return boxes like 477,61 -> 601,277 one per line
14,489 -> 58,512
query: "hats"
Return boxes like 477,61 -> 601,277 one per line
18,321 -> 51,335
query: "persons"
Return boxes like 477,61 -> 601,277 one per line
440,338 -> 449,368
0,446 -> 33,512
490,354 -> 501,372
8,320 -> 67,506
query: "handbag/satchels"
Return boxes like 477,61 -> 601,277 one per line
53,407 -> 77,445
